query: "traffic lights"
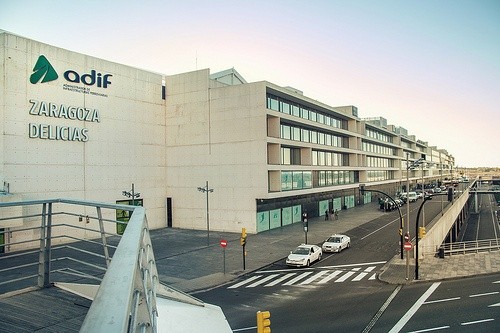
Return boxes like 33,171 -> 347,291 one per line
240,233 -> 248,247
257,310 -> 271,333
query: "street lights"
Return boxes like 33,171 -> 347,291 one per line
406,157 -> 423,240
122,183 -> 141,207
197,181 -> 214,247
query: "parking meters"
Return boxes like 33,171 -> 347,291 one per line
302,222 -> 308,245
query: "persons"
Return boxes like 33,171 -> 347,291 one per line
325,209 -> 329,220
331,209 -> 335,219
379,195 -> 388,212
335,208 -> 339,219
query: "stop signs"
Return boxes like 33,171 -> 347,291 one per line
220,238 -> 227,248
404,233 -> 410,243
403,242 -> 413,251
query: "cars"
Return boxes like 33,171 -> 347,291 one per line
285,244 -> 323,268
320,233 -> 351,253
379,175 -> 470,212
